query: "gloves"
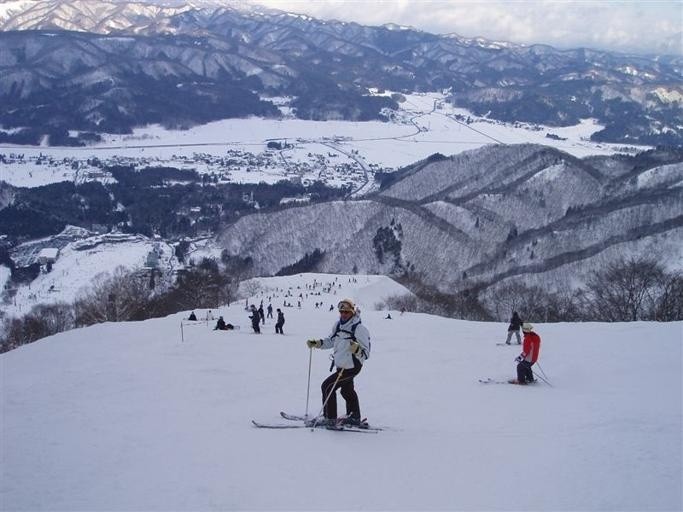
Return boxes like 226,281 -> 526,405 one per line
347,340 -> 362,355
308,339 -> 324,348
514,355 -> 522,362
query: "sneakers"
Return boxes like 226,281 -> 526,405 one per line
511,378 -> 534,384
310,415 -> 361,426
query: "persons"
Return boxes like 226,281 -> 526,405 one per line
307,299 -> 372,427
504,311 -> 524,344
513,324 -> 541,383
188,275 -> 408,336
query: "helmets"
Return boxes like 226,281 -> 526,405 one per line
525,324 -> 534,334
338,299 -> 356,316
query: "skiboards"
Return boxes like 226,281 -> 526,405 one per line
479,378 -> 538,384
251,411 -> 383,433
